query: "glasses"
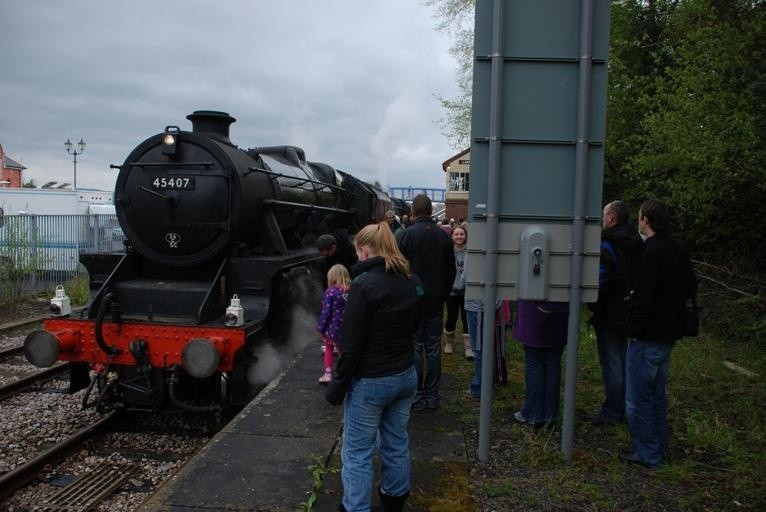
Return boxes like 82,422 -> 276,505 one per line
385,216 -> 395,219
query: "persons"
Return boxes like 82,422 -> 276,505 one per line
385,211 -> 402,237
316,264 -> 348,382
442,225 -> 477,358
511,300 -> 570,426
594,197 -> 645,429
326,222 -> 418,511
394,193 -> 457,411
433,215 -> 468,237
615,202 -> 703,470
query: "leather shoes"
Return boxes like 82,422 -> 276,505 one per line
377,485 -> 411,511
427,397 -> 440,410
339,502 -> 345,509
410,399 -> 424,412
335,370 -> 343,378
320,376 -> 333,383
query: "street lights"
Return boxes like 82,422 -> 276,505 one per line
65,139 -> 87,192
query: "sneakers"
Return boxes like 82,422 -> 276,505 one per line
515,410 -> 544,430
321,344 -> 338,355
618,451 -> 663,468
466,386 -> 482,396
589,413 -> 623,426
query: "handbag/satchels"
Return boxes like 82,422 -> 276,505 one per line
594,244 -> 629,333
681,246 -> 698,337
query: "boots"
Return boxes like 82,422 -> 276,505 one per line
444,330 -> 455,355
463,333 -> 475,361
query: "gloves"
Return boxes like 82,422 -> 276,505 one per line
324,374 -> 349,406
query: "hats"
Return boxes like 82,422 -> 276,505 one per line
316,233 -> 335,249
